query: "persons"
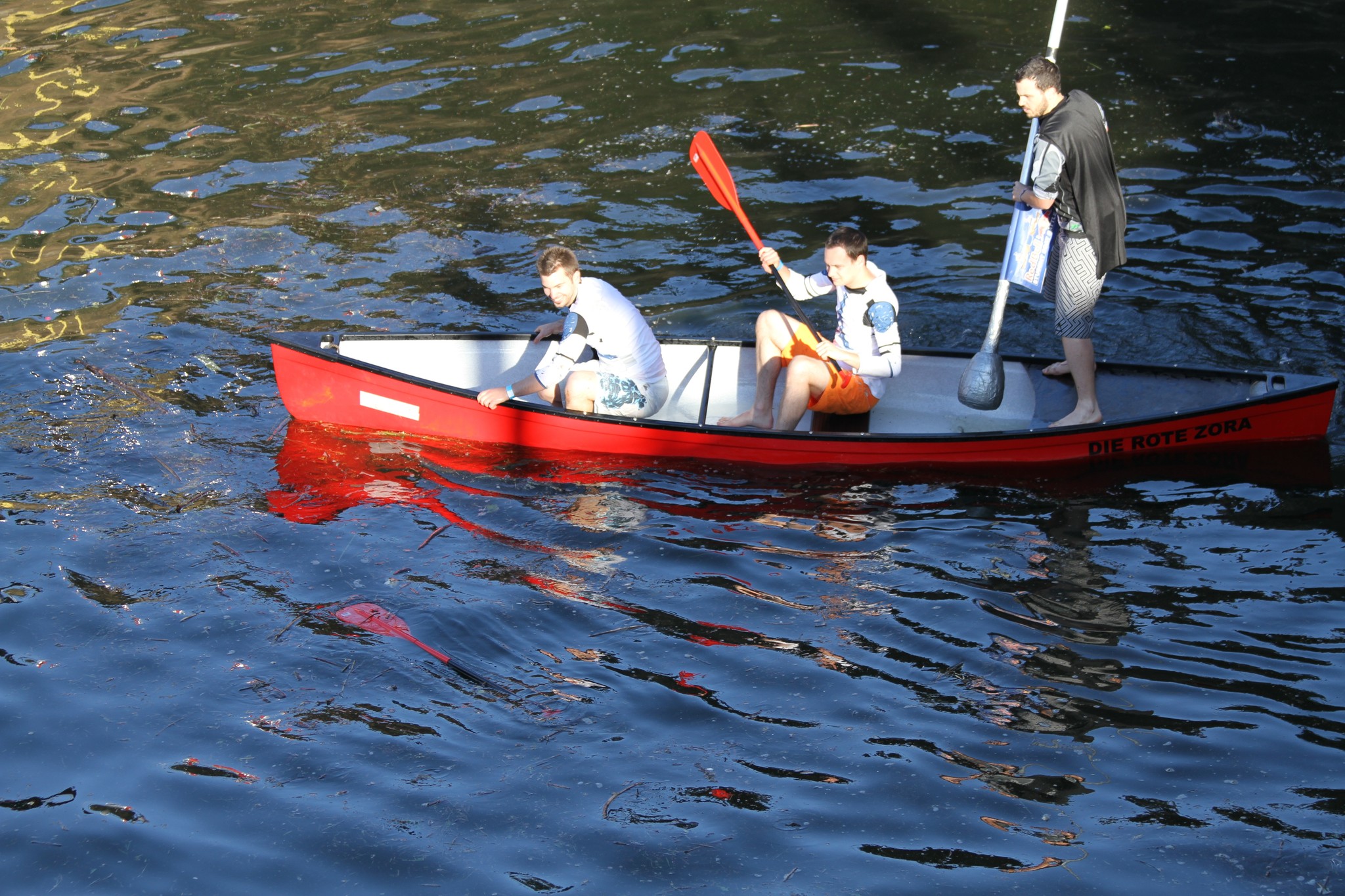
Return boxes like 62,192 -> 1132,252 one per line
1012,56 -> 1127,427
717,227 -> 902,428
477,246 -> 670,419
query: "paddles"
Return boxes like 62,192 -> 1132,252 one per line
689,128 -> 853,391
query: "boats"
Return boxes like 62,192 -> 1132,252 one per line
256,330 -> 1342,475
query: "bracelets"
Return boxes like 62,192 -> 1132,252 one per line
776,259 -> 783,270
506,385 -> 515,400
1019,187 -> 1030,203
